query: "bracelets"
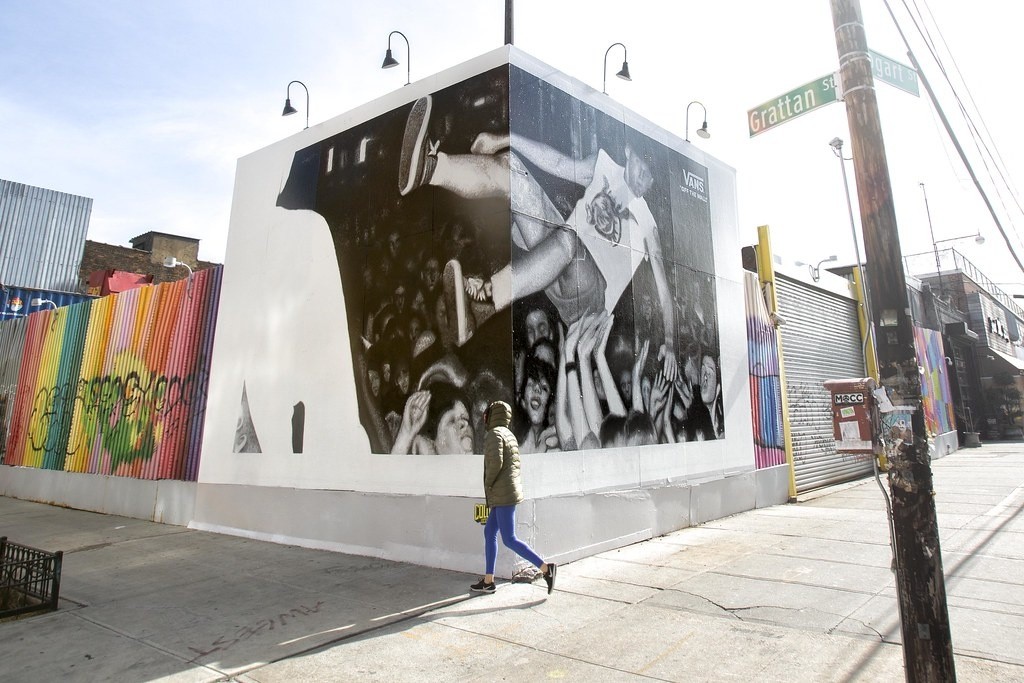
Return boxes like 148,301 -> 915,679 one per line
564,361 -> 577,375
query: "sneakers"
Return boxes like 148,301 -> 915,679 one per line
542,563 -> 556,595
444,261 -> 495,348
471,577 -> 496,593
398,95 -> 441,195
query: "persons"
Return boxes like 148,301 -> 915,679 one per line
469,400 -> 557,595
356,95 -> 725,455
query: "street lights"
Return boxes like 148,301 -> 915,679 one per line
932,230 -> 985,299
829,135 -> 878,378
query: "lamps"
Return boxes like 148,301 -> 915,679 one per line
162,256 -> 193,300
282,80 -> 310,130
685,101 -> 710,143
987,355 -> 995,360
944,356 -> 953,366
603,43 -> 632,96
382,31 -> 411,86
31,298 -> 58,332
809,255 -> 837,282
